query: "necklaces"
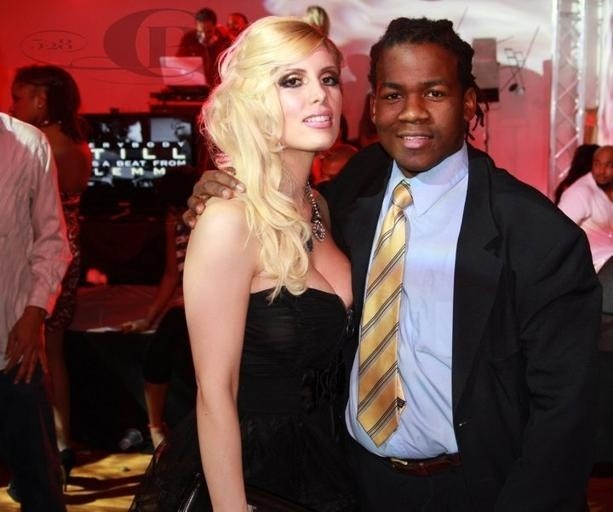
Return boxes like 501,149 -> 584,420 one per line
301,181 -> 328,250
41,117 -> 63,128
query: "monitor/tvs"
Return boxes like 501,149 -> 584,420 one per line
78,110 -> 205,212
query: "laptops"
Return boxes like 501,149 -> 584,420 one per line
159,56 -> 208,89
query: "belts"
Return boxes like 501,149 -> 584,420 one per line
389,456 -> 461,476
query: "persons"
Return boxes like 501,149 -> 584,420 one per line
118,162 -> 203,455
557,146 -> 613,316
183,18 -> 604,512
128,14 -> 357,512
217,11 -> 250,44
553,143 -> 600,205
8,64 -> 91,492
177,7 -> 231,90
0,113 -> 75,512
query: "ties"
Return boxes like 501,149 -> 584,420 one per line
357,180 -> 413,449
